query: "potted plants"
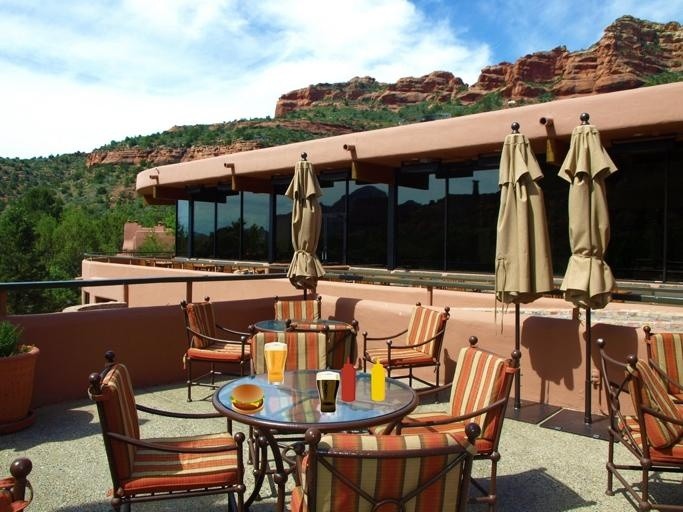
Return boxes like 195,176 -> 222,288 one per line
0,320 -> 40,436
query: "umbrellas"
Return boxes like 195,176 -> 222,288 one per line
559,125 -> 620,314
284,160 -> 328,297
497,132 -> 557,310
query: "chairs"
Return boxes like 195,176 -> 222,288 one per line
643,326 -> 683,414
596,338 -> 683,512
